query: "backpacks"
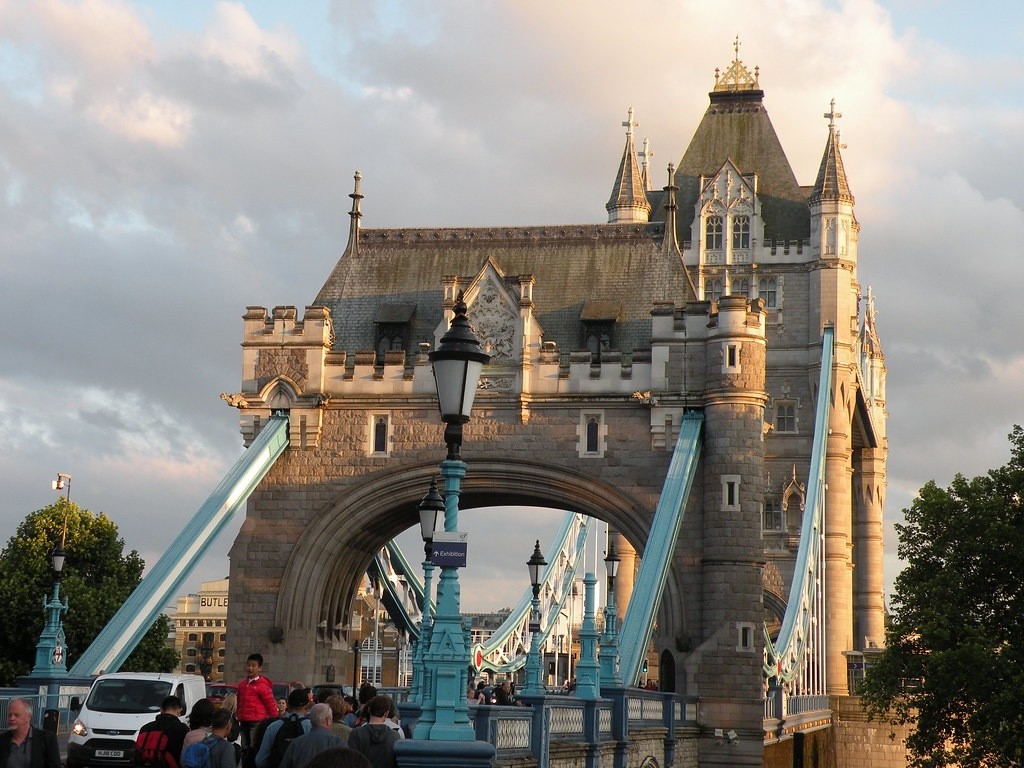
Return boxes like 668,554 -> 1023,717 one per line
270,713 -> 309,768
184,736 -> 223,768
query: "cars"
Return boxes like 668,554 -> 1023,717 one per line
206,682 -> 239,711
271,680 -> 306,704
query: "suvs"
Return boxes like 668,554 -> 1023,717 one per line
312,684 -> 361,706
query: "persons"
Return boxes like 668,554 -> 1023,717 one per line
236,653 -> 279,753
468,681 -> 522,707
0,698 -> 62,768
644,679 -> 656,691
568,679 -> 576,690
135,693 -> 241,768
245,678 -> 406,768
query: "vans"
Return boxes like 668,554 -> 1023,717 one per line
66,671 -> 207,768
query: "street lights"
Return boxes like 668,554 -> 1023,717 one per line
599,539 -> 622,689
396,472 -> 446,738
520,538 -> 548,695
51,472 -> 72,549
31,537 -> 69,678
393,285 -> 498,768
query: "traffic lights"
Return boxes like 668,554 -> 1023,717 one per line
643,659 -> 648,673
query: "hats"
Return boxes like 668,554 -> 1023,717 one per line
288,689 -> 308,706
